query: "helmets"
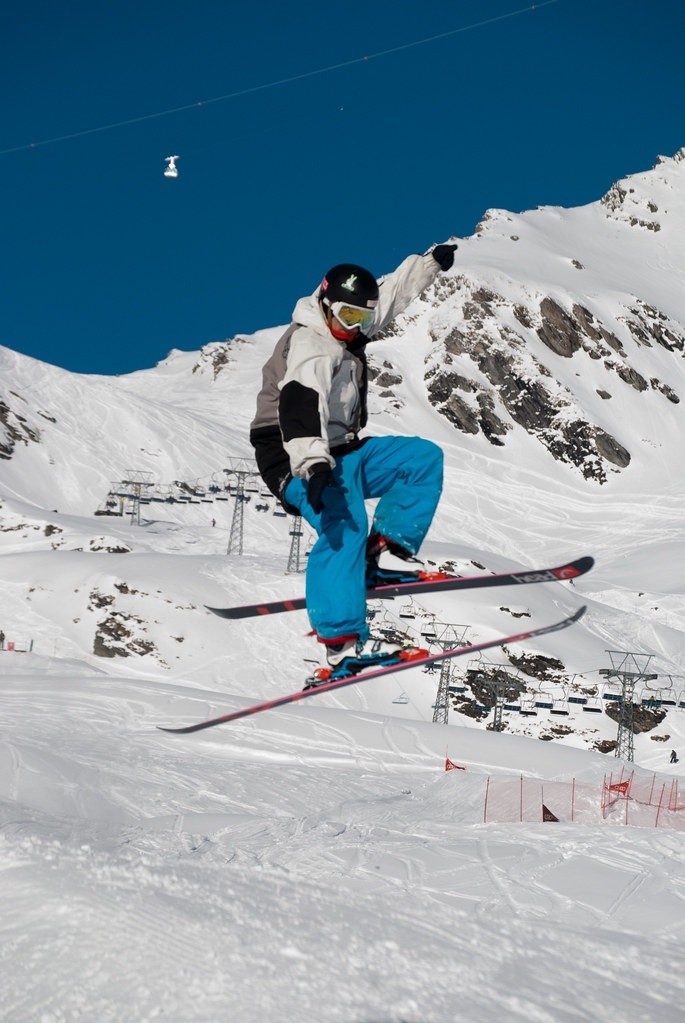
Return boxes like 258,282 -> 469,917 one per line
320,264 -> 379,314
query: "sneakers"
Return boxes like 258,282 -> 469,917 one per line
324,635 -> 404,668
365,533 -> 437,585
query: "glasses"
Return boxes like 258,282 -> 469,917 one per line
332,302 -> 376,332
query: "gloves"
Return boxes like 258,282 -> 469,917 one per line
307,463 -> 337,514
433,245 -> 458,272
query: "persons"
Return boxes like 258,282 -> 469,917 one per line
249,243 -> 458,683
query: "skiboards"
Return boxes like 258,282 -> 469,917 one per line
150,554 -> 597,739
